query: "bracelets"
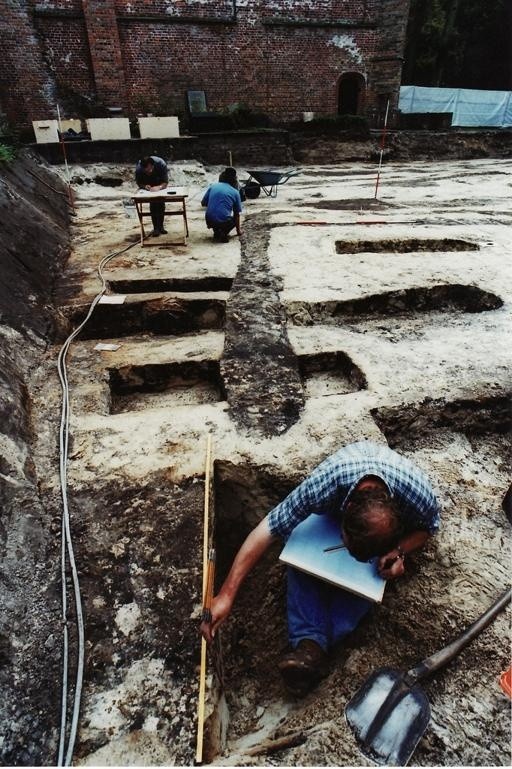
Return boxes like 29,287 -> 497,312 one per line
396,545 -> 408,562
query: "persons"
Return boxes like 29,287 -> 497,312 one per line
199,441 -> 440,674
135,155 -> 169,236
226,169 -> 246,202
201,172 -> 244,242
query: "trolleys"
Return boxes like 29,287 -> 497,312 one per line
247,170 -> 301,198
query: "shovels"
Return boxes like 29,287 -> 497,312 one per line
345,589 -> 512,767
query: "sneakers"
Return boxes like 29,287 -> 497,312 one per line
280,638 -> 326,697
160,229 -> 168,234
152,230 -> 160,237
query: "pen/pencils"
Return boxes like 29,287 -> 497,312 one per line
324,544 -> 344,551
373,555 -> 400,579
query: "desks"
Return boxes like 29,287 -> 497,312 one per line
130,186 -> 189,247
